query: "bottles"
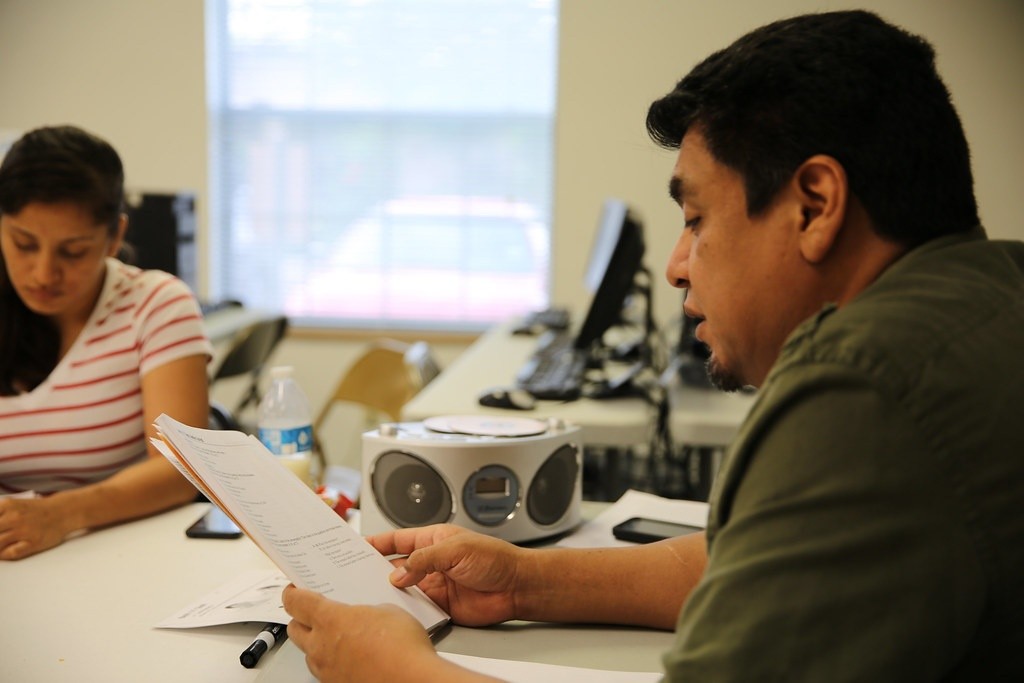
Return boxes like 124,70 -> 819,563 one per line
259,367 -> 311,489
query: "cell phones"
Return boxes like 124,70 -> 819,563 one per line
186,505 -> 244,539
612,515 -> 706,545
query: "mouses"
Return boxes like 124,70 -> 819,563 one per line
480,387 -> 537,411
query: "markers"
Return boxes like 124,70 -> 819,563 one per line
238,623 -> 288,669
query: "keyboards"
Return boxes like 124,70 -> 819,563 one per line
512,309 -> 585,401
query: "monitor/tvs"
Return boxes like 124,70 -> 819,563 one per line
570,199 -> 663,401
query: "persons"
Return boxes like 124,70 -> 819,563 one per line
283,12 -> 1024,683
0,124 -> 213,561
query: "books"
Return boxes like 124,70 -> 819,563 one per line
146,414 -> 453,642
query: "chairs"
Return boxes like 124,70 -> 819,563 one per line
310,346 -> 420,435
204,319 -> 291,433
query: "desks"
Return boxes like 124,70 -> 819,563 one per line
402,324 -> 757,503
1,501 -> 676,683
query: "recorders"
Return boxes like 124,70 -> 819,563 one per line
359,412 -> 583,544
